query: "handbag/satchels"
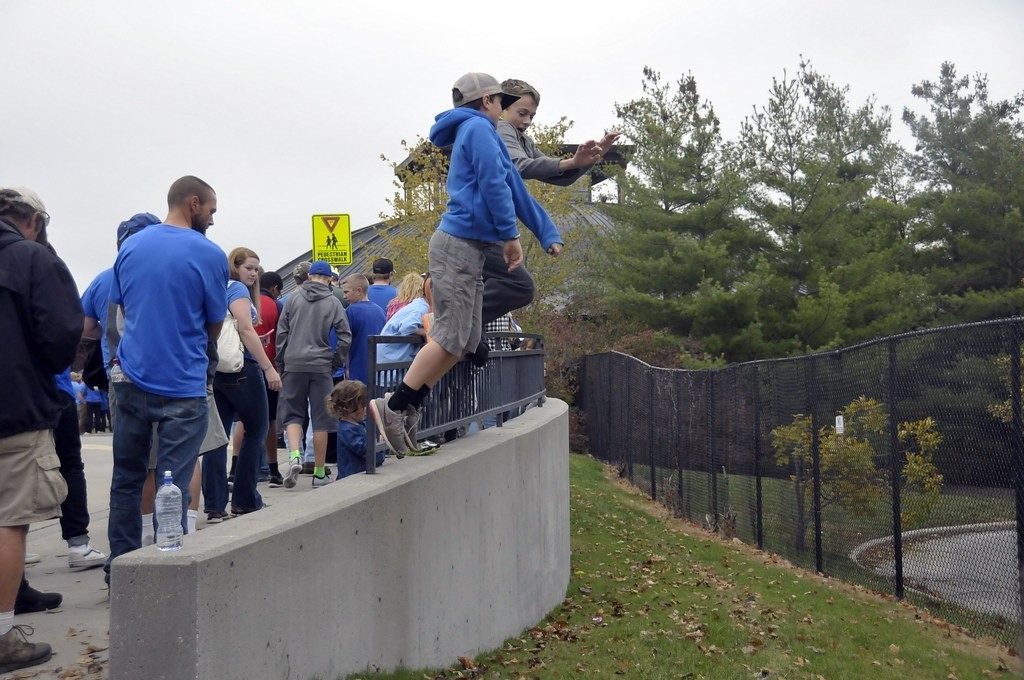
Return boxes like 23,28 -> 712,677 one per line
216,281 -> 244,374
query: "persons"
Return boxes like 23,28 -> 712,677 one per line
14,577 -> 62,613
52,366 -> 109,570
421,80 -> 622,368
81,176 -> 545,597
1,183 -> 86,673
68,369 -> 113,435
367,70 -> 565,456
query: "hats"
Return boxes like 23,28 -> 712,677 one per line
309,261 -> 332,277
452,73 -> 521,110
0,185 -> 48,227
373,258 -> 398,274
117,222 -> 129,244
119,212 -> 161,241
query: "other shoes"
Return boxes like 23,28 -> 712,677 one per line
83,431 -> 104,437
302,462 -> 331,475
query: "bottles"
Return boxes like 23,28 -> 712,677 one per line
153,470 -> 184,551
110,358 -> 125,382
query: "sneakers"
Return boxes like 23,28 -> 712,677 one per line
405,443 -> 434,456
227,474 -> 235,493
283,457 -> 302,488
230,506 -> 243,516
68,541 -> 109,573
0,625 -> 53,674
268,473 -> 284,487
424,439 -> 441,454
312,475 -> 334,488
402,403 -> 422,452
14,571 -> 62,615
369,392 -> 409,457
206,510 -> 228,523
24,553 -> 38,563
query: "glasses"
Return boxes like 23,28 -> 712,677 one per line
41,211 -> 51,226
273,282 -> 282,300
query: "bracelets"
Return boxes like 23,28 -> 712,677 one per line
513,233 -> 521,240
264,363 -> 273,372
